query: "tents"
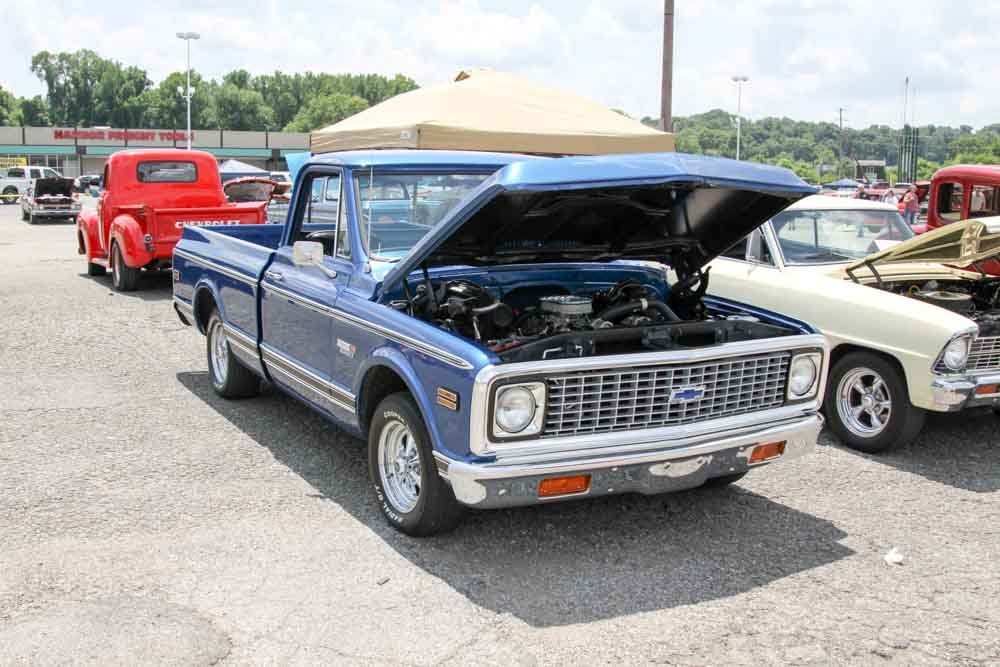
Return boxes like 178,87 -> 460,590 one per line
823,178 -> 869,190
218,159 -> 272,180
307,68 -> 675,224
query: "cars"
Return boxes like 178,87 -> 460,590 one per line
74,174 -> 104,194
0,166 -> 65,205
639,191 -> 1000,452
265,150 -> 443,233
22,175 -> 82,224
873,165 -> 1000,281
172,145 -> 835,538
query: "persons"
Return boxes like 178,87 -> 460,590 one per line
884,190 -> 897,205
856,184 -> 871,200
903,184 -> 919,225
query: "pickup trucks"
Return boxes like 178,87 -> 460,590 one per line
76,147 -> 270,292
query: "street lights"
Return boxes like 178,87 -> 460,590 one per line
177,30 -> 201,153
731,74 -> 751,163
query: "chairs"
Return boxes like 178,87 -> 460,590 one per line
305,229 -> 336,258
